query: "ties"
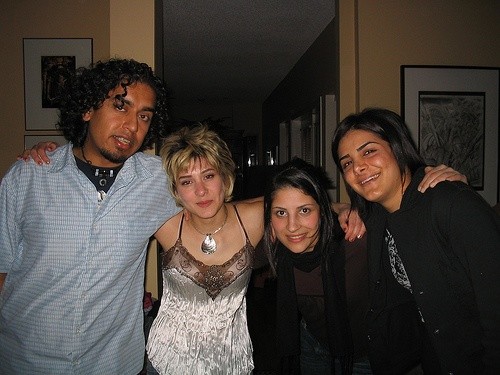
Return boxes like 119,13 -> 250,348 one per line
384,230 -> 411,293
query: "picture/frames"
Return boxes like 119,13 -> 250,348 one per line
22,36 -> 94,132
400,63 -> 500,207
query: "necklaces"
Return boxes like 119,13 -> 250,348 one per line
189,204 -> 228,254
81,145 -> 124,187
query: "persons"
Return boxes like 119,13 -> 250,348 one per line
270,106 -> 500,375
0,57 -> 184,375
14,121 -> 367,375
184,157 -> 468,375
